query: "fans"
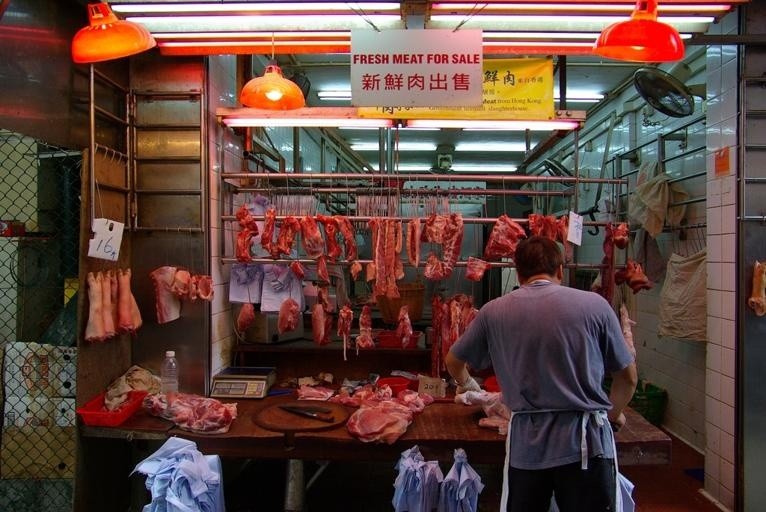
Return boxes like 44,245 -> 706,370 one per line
540,156 -> 589,191
633,61 -> 706,118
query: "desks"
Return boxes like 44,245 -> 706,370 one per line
79,390 -> 672,511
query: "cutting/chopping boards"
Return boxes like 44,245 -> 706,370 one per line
251,401 -> 349,433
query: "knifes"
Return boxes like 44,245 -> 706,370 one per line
278,404 -> 334,422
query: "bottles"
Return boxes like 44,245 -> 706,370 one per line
161,351 -> 179,395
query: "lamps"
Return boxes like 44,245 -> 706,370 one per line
239,30 -> 306,111
216,105 -> 586,131
69,3 -> 156,64
593,1 -> 684,61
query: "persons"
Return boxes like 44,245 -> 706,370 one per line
441,233 -> 640,512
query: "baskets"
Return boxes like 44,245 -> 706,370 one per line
376,262 -> 427,324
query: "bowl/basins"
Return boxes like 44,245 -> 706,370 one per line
376,375 -> 411,396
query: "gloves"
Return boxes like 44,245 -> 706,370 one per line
456,378 -> 482,395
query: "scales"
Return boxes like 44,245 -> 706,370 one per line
210,367 -> 278,398
